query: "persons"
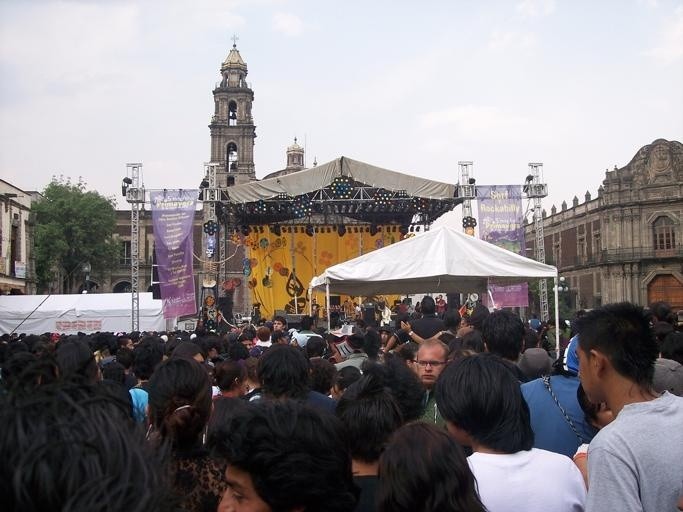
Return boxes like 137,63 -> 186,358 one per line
1,289 -> 683,511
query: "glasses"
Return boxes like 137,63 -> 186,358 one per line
415,360 -> 447,368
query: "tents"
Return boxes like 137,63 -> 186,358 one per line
308,226 -> 559,361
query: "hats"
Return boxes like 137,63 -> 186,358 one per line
516,348 -> 556,380
651,358 -> 683,395
